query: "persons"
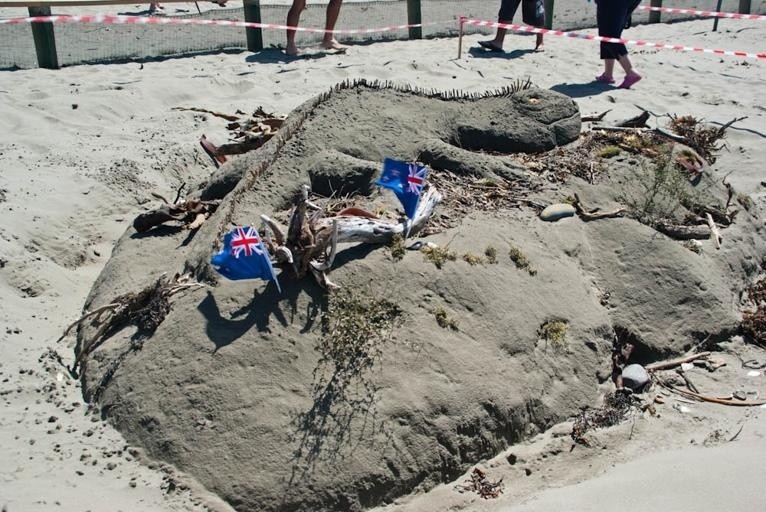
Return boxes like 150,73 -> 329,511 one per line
595,1 -> 642,90
475,1 -> 553,52
283,1 -> 348,57
149,2 -> 165,13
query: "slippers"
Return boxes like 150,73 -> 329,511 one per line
478,40 -> 502,50
595,72 -> 615,83
619,72 -> 641,89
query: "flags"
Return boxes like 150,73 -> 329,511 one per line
368,156 -> 432,224
209,222 -> 279,287
